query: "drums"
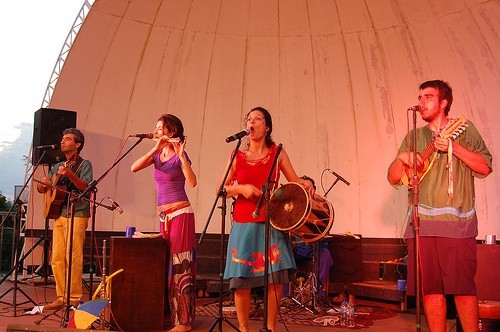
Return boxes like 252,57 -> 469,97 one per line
265,182 -> 336,244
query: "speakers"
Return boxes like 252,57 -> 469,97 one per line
32,107 -> 78,166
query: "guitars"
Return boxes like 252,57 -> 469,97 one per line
403,112 -> 469,184
41,153 -> 78,219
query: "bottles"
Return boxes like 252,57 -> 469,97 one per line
340,297 -> 355,327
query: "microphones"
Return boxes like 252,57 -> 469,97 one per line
130,133 -> 154,139
408,105 -> 422,111
327,168 -> 350,186
225,128 -> 251,143
36,145 -> 55,149
107,196 -> 123,214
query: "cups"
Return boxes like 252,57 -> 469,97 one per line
397,279 -> 406,290
126,226 -> 135,238
484,234 -> 496,245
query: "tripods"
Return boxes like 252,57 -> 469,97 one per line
1,150 -> 115,328
199,139 -> 242,332
281,242 -> 339,316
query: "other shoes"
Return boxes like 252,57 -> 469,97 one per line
62,300 -> 79,311
280,295 -> 295,309
45,298 -> 65,310
315,295 -> 332,307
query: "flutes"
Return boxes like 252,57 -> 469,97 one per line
152,138 -> 183,143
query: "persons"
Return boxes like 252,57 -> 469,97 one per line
387,80 -> 493,332
283,175 -> 333,306
130,113 -> 197,332
37,128 -> 93,312
215,107 -> 316,332
